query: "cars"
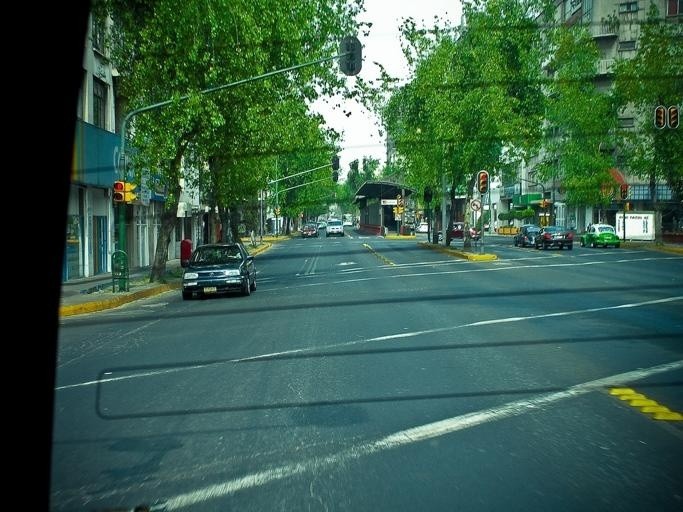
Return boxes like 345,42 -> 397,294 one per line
300,220 -> 344,238
534,225 -> 573,250
404,221 -> 431,233
179,242 -> 257,300
512,224 -> 541,247
580,223 -> 619,248
436,221 -> 480,242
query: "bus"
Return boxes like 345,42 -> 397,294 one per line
342,213 -> 353,226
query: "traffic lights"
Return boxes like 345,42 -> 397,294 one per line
124,182 -> 137,202
619,184 -> 627,199
625,202 -> 632,211
113,181 -> 124,202
543,201 -> 548,209
477,170 -> 489,195
392,207 -> 397,214
398,207 -> 403,214
539,200 -> 543,208
654,106 -> 667,129
396,195 -> 401,206
668,106 -> 679,128
